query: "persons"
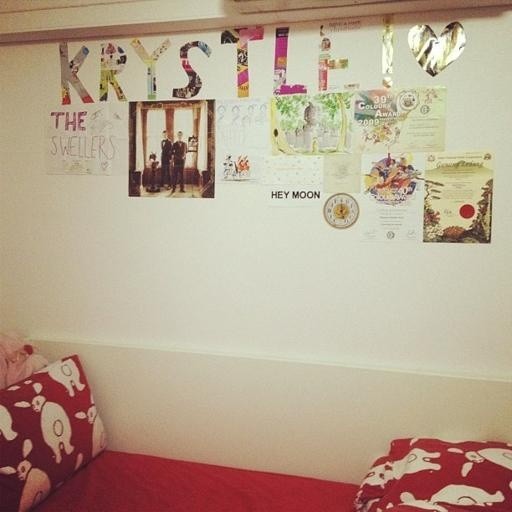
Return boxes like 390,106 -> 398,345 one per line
232,105 -> 246,124
146,153 -> 160,193
216,104 -> 229,124
259,105 -> 267,117
158,130 -> 172,190
171,132 -> 187,192
241,105 -> 256,120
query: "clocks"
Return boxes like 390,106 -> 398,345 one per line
323,192 -> 359,229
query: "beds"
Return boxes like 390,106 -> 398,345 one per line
26,448 -> 360,512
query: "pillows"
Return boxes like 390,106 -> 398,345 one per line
0,355 -> 107,512
